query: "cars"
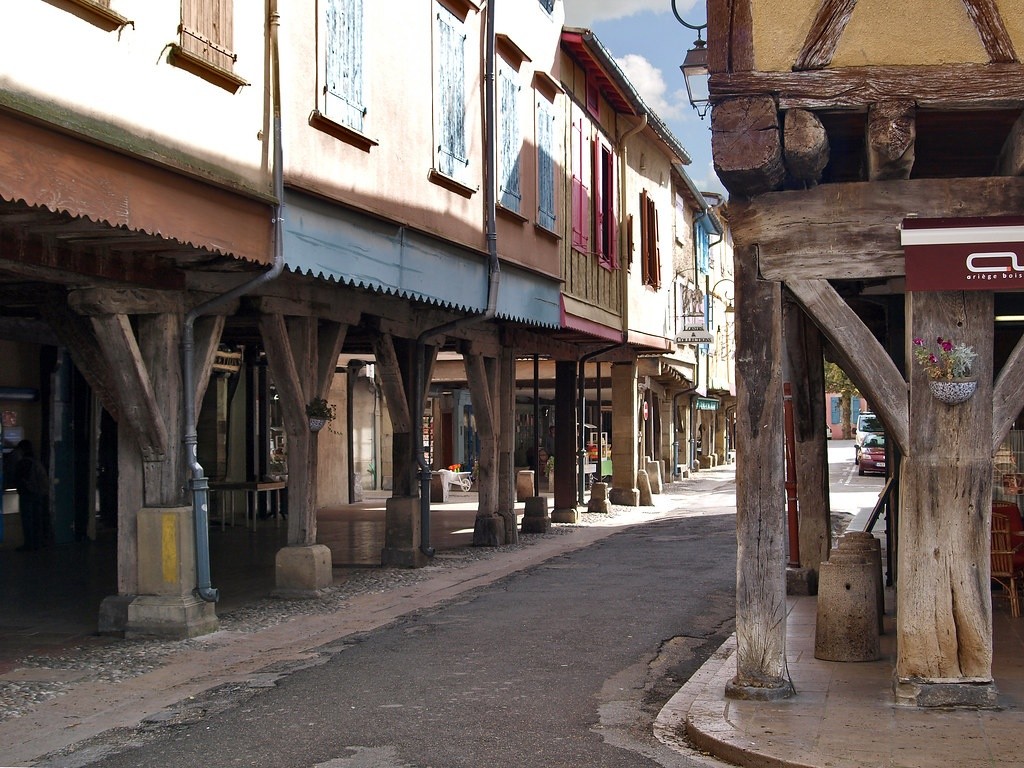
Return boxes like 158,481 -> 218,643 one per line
827,424 -> 832,440
854,432 -> 885,476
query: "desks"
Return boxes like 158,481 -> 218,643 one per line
205,479 -> 284,531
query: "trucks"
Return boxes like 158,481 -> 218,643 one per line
855,412 -> 885,464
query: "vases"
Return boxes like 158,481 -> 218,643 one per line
930,378 -> 976,405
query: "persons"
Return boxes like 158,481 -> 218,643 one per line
544,426 -> 555,456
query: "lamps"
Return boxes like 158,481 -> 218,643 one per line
671,0 -> 715,121
710,279 -> 735,330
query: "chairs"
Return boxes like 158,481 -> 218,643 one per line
988,447 -> 1023,621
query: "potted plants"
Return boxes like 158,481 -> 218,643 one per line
306,397 -> 343,436
544,457 -> 555,493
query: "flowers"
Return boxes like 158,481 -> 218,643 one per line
914,338 -> 977,379
